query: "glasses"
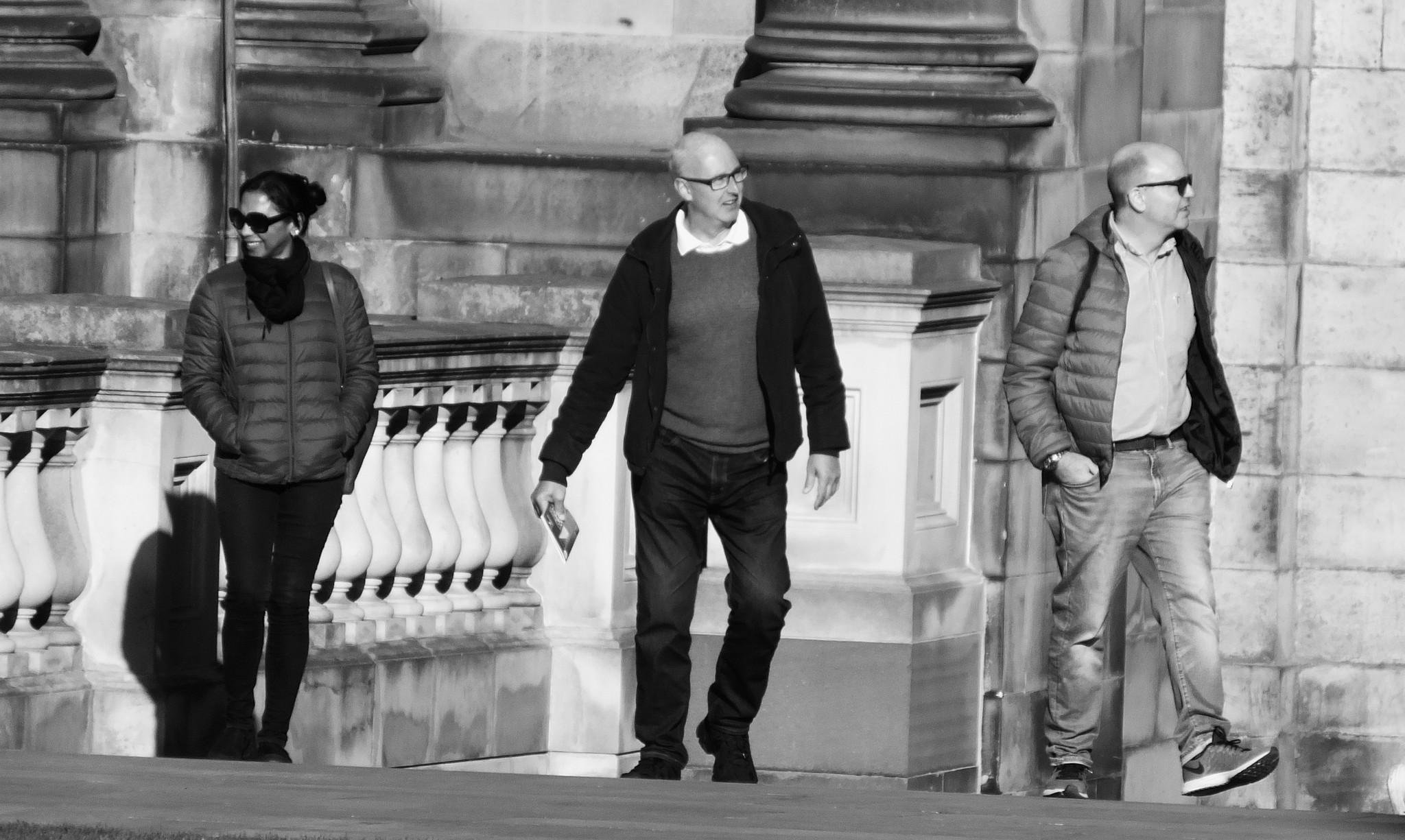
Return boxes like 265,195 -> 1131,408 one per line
1137,174 -> 1193,196
228,206 -> 290,234
679,161 -> 749,191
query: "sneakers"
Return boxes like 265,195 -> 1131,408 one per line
621,755 -> 681,781
209,714 -> 256,760
1042,762 -> 1090,799
257,741 -> 294,764
1181,726 -> 1280,797
695,714 -> 758,784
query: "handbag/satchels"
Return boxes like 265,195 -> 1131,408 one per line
341,407 -> 378,494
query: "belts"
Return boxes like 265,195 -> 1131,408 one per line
1111,424 -> 1186,451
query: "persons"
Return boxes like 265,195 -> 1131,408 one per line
530,132 -> 851,786
1000,141 -> 1279,800
180,171 -> 380,763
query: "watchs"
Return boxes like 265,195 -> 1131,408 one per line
1044,448 -> 1068,472
